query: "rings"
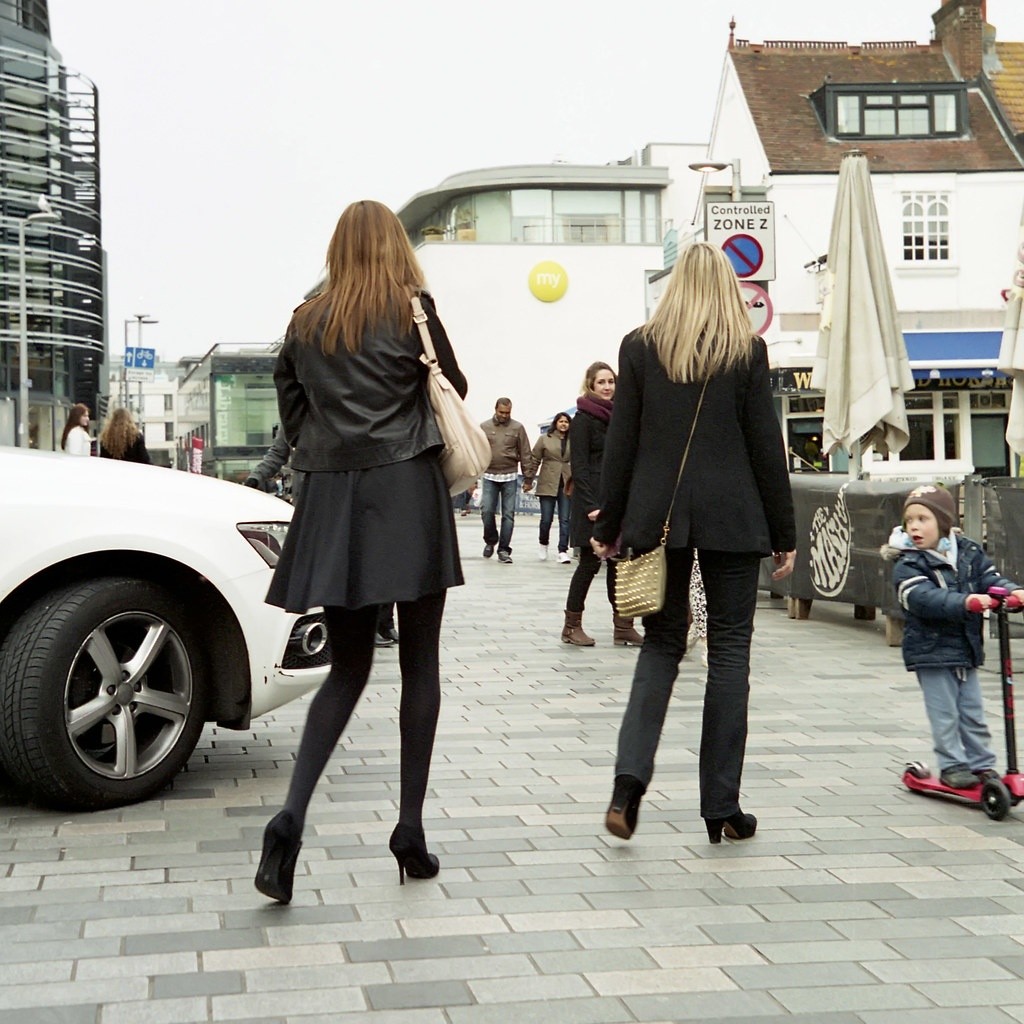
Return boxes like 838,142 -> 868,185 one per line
772,552 -> 783,557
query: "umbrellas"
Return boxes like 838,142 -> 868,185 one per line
996,195 -> 1024,460
808,148 -> 915,482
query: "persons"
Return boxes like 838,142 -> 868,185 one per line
254,200 -> 467,902
561,361 -> 643,646
879,484 -> 1024,790
460,480 -> 477,516
61,404 -> 91,456
480,397 -> 533,563
245,423 -> 399,648
590,237 -> 796,842
100,407 -> 153,466
522,412 -> 572,565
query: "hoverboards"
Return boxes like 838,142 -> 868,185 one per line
901,585 -> 1024,823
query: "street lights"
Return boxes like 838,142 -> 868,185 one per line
133,313 -> 151,434
18,212 -> 63,448
123,319 -> 163,409
689,156 -> 742,202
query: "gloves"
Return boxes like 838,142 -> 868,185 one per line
245,478 -> 258,487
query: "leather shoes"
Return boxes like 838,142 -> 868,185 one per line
374,633 -> 394,646
384,628 -> 400,643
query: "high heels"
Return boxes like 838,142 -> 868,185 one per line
389,823 -> 439,885
255,810 -> 302,902
605,774 -> 644,839
703,808 -> 758,843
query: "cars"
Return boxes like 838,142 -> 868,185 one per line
0,443 -> 335,817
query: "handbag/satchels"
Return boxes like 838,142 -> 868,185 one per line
410,296 -> 492,497
615,545 -> 667,615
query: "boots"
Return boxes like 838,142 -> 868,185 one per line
561,611 -> 594,646
613,614 -> 644,645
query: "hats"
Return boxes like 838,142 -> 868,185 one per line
902,481 -> 955,551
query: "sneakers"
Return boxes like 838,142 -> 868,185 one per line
939,763 -> 980,788
497,551 -> 512,563
557,552 -> 571,563
976,769 -> 1001,782
539,544 -> 548,560
484,537 -> 498,557
